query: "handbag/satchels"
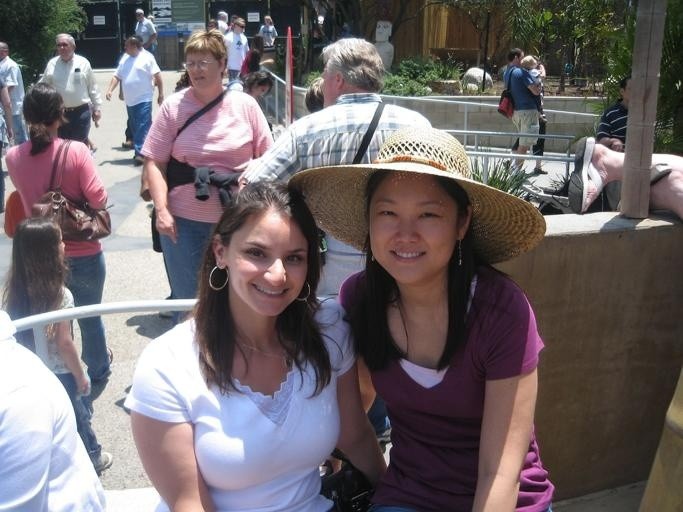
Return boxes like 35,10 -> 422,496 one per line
30,137 -> 112,243
316,446 -> 378,512
496,89 -> 516,120
317,228 -> 329,268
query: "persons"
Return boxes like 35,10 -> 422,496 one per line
1,216 -> 116,473
521,55 -> 547,120
206,9 -> 324,139
119,35 -> 157,148
123,180 -> 388,511
3,82 -> 116,384
233,35 -> 434,296
499,111 -> 548,176
501,48 -> 543,181
0,41 -> 28,147
1,308 -> 110,512
36,34 -> 103,145
139,27 -> 275,328
133,8 -> 159,56
105,36 -> 163,164
0,62 -> 16,211
286,125 -> 557,512
596,75 -> 632,210
566,136 -> 683,222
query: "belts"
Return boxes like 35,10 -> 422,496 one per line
63,101 -> 92,113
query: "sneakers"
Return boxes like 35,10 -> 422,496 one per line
158,308 -> 179,318
90,345 -> 114,383
533,166 -> 549,175
121,141 -> 135,149
133,152 -> 146,165
91,450 -> 115,473
502,158 -> 516,175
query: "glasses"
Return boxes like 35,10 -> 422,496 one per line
56,43 -> 71,47
182,60 -> 219,71
234,22 -> 246,29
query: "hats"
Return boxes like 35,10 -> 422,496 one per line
285,124 -> 548,268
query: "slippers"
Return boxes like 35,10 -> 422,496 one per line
616,161 -> 673,214
567,134 -> 605,216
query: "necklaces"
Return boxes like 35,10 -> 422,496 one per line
237,338 -> 292,371
